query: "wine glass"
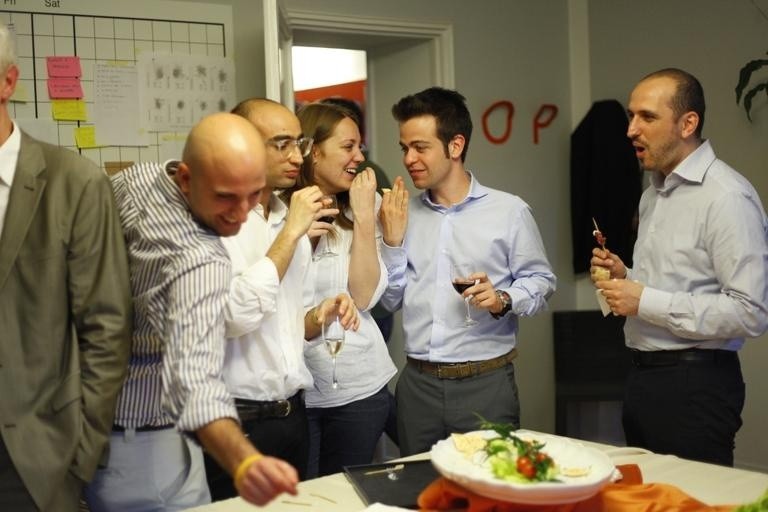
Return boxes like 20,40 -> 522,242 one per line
450,262 -> 480,327
322,303 -> 345,390
316,194 -> 339,258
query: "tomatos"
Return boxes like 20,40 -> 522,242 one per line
516,453 -> 545,478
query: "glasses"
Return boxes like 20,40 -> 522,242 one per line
264,137 -> 314,157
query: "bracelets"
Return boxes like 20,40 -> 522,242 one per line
232,454 -> 267,491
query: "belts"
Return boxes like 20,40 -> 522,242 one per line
111,424 -> 176,433
235,389 -> 303,420
420,349 -> 518,380
632,349 -> 737,366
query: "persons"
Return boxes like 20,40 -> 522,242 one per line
589,68 -> 768,468
191,97 -> 362,501
0,24 -> 134,512
379,87 -> 557,460
272,97 -> 400,482
83,111 -> 301,512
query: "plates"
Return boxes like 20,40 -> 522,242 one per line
429,429 -> 616,506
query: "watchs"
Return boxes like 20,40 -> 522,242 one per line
488,289 -> 513,320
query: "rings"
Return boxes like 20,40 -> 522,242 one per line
318,200 -> 326,208
